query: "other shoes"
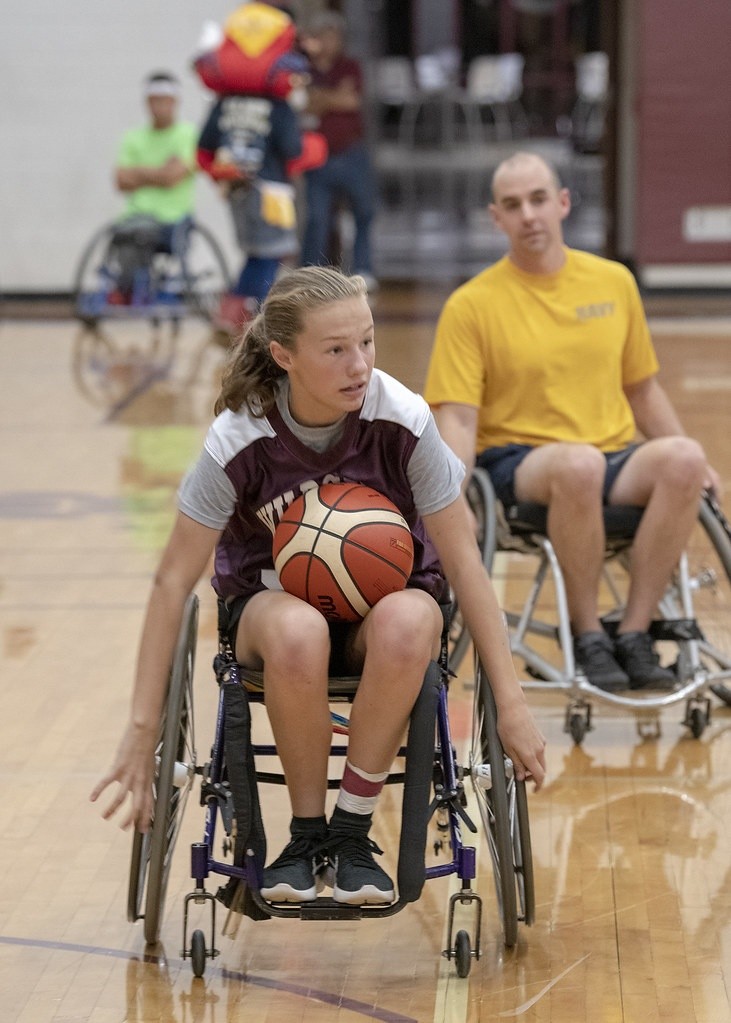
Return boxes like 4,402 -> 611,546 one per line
574,631 -> 629,688
616,631 -> 675,689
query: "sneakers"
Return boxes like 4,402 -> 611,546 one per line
324,833 -> 396,904
260,830 -> 329,902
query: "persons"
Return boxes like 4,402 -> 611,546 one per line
105,0 -> 378,347
425,153 -> 722,693
87,267 -> 545,904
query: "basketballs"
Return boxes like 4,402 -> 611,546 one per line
270,481 -> 416,623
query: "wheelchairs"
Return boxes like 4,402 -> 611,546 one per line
71,212 -> 254,348
443,465 -> 731,740
127,557 -> 536,976
